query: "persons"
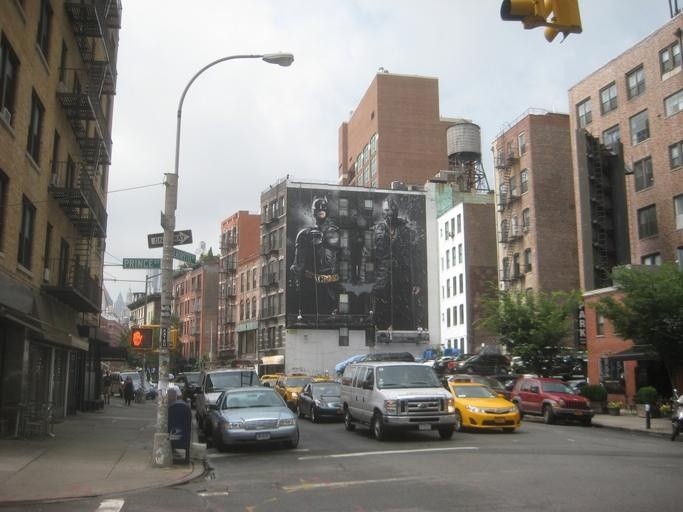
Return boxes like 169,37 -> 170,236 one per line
288,193 -> 339,316
168,373 -> 182,401
357,196 -> 424,330
102,370 -> 112,405
122,376 -> 132,407
669,395 -> 682,440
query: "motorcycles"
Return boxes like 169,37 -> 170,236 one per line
671,389 -> 683,441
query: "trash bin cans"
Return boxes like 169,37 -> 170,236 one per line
168,400 -> 191,465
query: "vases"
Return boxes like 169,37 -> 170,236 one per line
608,409 -> 620,415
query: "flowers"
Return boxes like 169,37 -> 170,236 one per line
607,400 -> 624,408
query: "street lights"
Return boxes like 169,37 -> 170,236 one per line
143,267 -> 194,373
151,52 -> 293,469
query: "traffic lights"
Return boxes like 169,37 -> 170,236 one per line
500,0 -> 583,44
130,328 -> 153,350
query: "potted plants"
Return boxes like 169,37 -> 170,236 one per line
635,386 -> 657,418
581,384 -> 608,414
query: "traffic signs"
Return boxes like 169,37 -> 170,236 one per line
147,229 -> 194,249
